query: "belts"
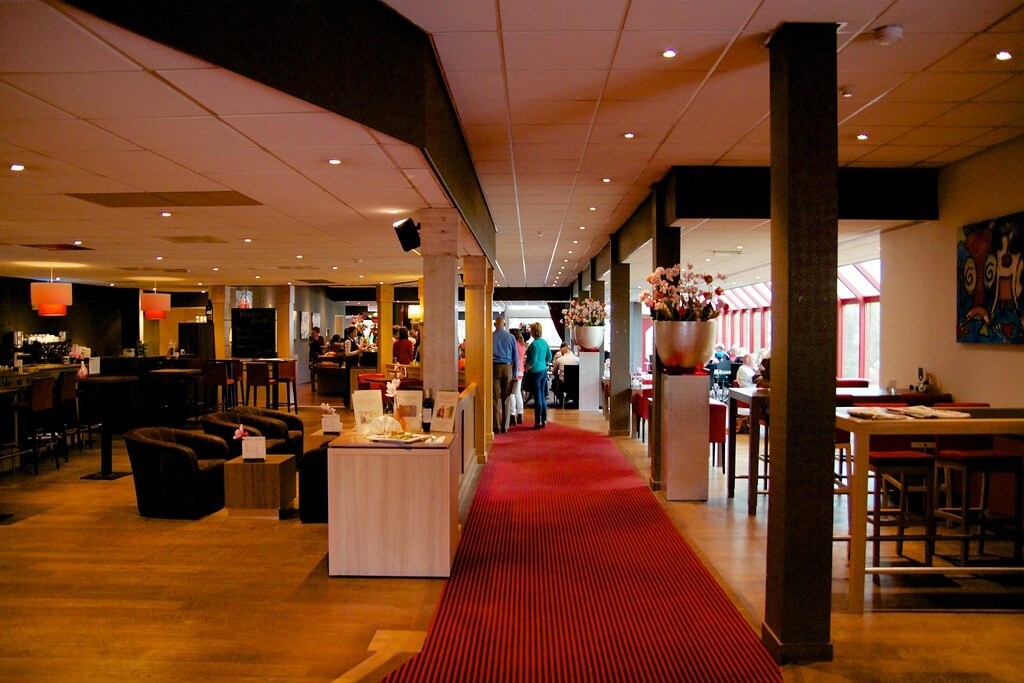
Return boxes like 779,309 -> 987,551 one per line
493,362 -> 511,363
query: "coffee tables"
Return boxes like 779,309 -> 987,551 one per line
224,454 -> 297,509
310,428 -> 351,449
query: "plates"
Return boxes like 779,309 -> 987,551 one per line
366,431 -> 433,444
320,346 -> 328,347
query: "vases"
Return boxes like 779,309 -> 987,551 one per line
521,333 -> 531,342
394,406 -> 405,434
241,436 -> 265,463
575,325 -> 603,348
322,413 -> 343,435
652,318 -> 718,371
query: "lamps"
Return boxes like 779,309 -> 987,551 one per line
30,266 -> 171,320
843,90 -> 853,98
408,305 -> 422,322
458,286 -> 465,302
391,217 -> 421,253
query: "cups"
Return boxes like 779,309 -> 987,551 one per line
181,349 -> 185,354
62,356 -> 70,364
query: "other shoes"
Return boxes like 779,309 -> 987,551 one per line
493,428 -> 507,433
529,425 -> 540,429
541,422 -> 546,428
312,384 -> 316,391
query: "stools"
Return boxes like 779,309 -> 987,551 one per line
11,359 -> 298,476
649,354 -> 1024,586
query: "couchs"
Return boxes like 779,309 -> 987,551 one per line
122,405 -> 329,524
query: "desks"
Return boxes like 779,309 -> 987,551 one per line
366,377 -> 419,401
74,358 -> 288,479
599,371 -> 1024,615
327,431 -> 458,578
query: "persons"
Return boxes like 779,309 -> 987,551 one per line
392,324 -> 420,365
525,322 -> 552,430
508,328 -> 526,426
709,342 -> 771,388
492,315 -> 519,434
330,334 -> 344,353
551,342 -> 579,405
342,326 -> 367,410
308,326 -> 324,392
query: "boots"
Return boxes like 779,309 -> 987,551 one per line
518,414 -> 523,424
509,414 -> 517,425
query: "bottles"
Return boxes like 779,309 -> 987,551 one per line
77,361 -> 88,378
384,397 -> 393,413
422,389 -> 434,432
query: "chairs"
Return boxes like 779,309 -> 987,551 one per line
557,365 -> 579,410
358,373 -> 423,411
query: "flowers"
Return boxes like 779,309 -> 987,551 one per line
385,379 -> 401,406
560,298 -> 608,327
641,263 -> 730,322
518,321 -> 530,333
352,315 -> 368,338
320,401 -> 336,415
232,424 -> 250,440
